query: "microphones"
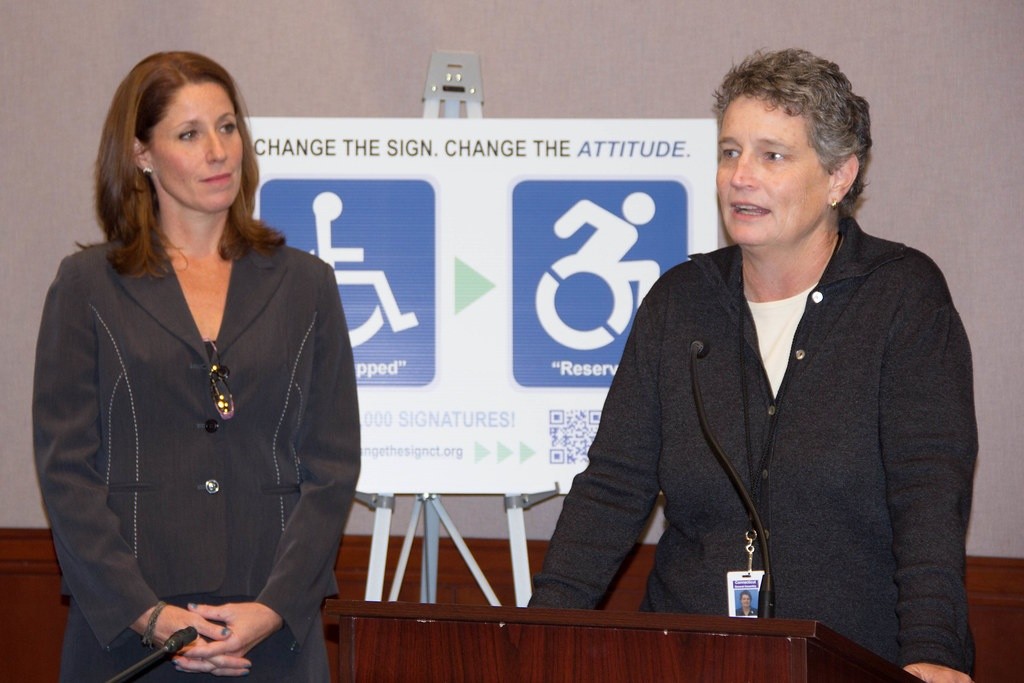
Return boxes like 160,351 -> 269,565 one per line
689,336 -> 777,617
106,626 -> 198,682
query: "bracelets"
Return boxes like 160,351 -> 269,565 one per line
142,600 -> 166,651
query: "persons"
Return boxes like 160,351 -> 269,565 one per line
526,48 -> 980,683
33,50 -> 362,683
